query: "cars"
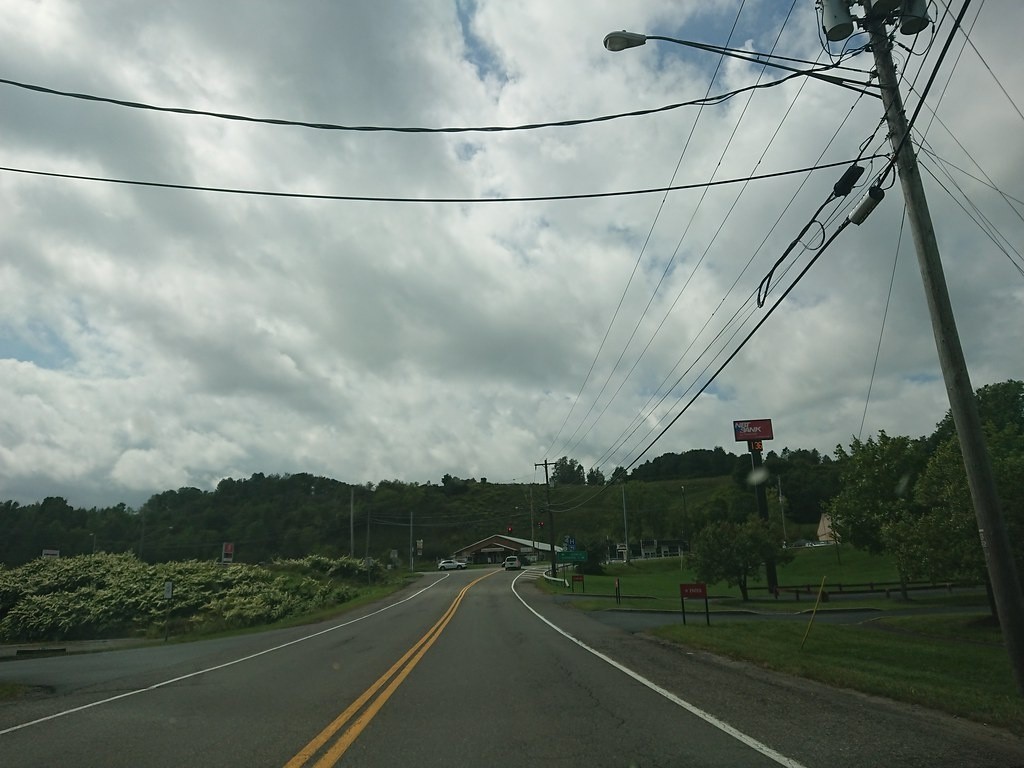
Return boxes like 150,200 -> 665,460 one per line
505,555 -> 522,570
437,559 -> 467,570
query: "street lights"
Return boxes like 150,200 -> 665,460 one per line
603,27 -> 1024,690
